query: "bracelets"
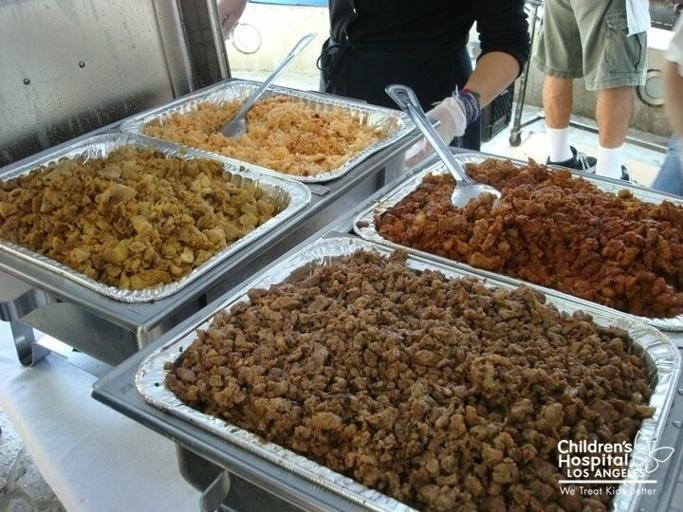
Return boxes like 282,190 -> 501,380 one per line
449,89 -> 481,128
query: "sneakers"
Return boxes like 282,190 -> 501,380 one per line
545,146 -> 597,174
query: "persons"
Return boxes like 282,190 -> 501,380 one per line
649,7 -> 683,199
214,0 -> 531,153
531,0 -> 651,182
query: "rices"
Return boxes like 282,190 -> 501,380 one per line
141,96 -> 380,176
